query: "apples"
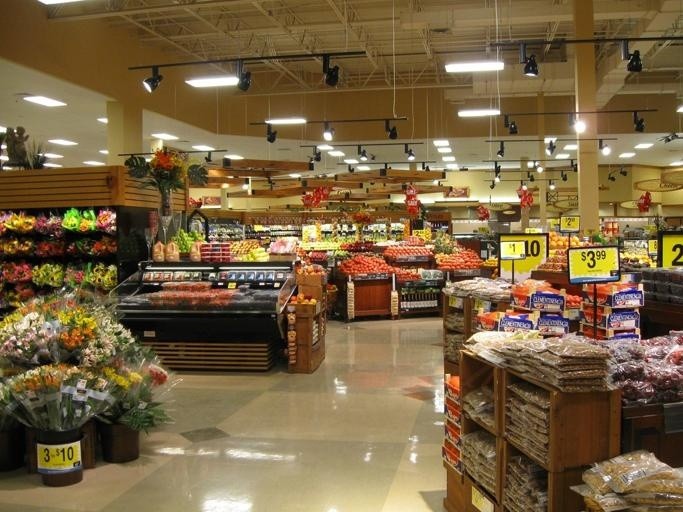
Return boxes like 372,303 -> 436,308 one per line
171,228 -> 208,254
290,264 -> 325,306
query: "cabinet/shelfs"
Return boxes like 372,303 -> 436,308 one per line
206,219 -> 485,321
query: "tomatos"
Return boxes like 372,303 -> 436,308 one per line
339,255 -> 422,283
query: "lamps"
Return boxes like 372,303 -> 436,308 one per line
124,48 -> 367,99
494,107 -> 660,135
486,30 -> 681,78
115,131 -> 683,192
246,114 -> 407,143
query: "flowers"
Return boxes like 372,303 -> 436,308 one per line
0,207 -> 169,439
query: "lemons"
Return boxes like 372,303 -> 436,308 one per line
546,232 -> 584,248
